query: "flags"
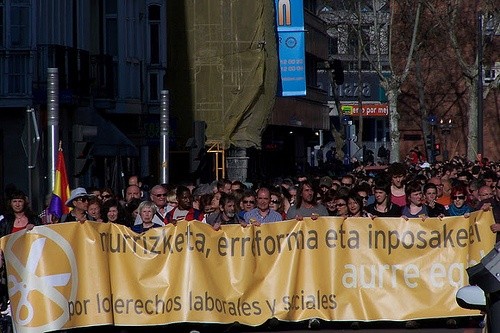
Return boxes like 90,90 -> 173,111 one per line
47,151 -> 73,217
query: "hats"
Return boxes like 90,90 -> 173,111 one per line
64,187 -> 97,207
333,179 -> 341,186
318,176 -> 333,189
282,178 -> 294,185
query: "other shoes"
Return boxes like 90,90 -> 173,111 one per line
308,318 -> 321,330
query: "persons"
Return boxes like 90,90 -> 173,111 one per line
0,174 -> 332,333
315,146 -> 500,333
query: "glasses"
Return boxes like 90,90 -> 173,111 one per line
361,195 -> 369,200
152,193 -> 167,197
334,202 -> 346,207
75,196 -> 90,203
270,199 -> 280,204
341,183 -> 352,187
243,200 -> 256,204
101,195 -> 111,200
452,196 -> 465,200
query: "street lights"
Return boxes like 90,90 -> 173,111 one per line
439,118 -> 457,152
